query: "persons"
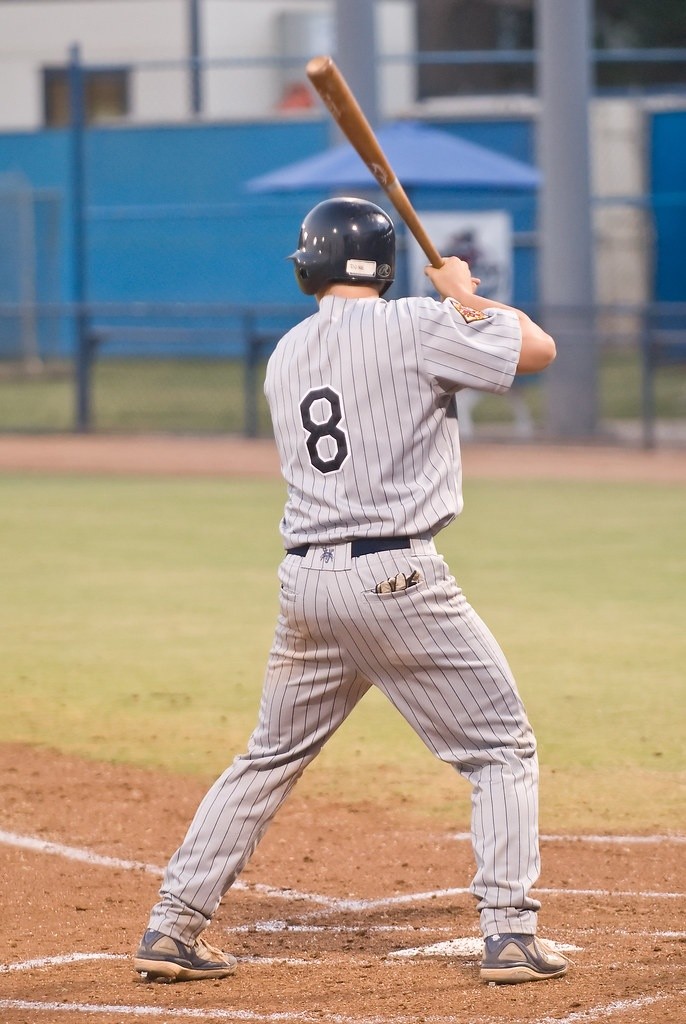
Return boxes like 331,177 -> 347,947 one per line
135,196 -> 569,981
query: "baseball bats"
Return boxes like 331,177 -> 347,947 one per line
306,54 -> 447,271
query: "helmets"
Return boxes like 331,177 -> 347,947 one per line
287,196 -> 396,297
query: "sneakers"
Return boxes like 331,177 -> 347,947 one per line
131,929 -> 237,979
480,932 -> 576,980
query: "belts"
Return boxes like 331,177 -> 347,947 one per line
287,537 -> 410,559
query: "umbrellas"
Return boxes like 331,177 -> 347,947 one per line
247,124 -> 542,298
